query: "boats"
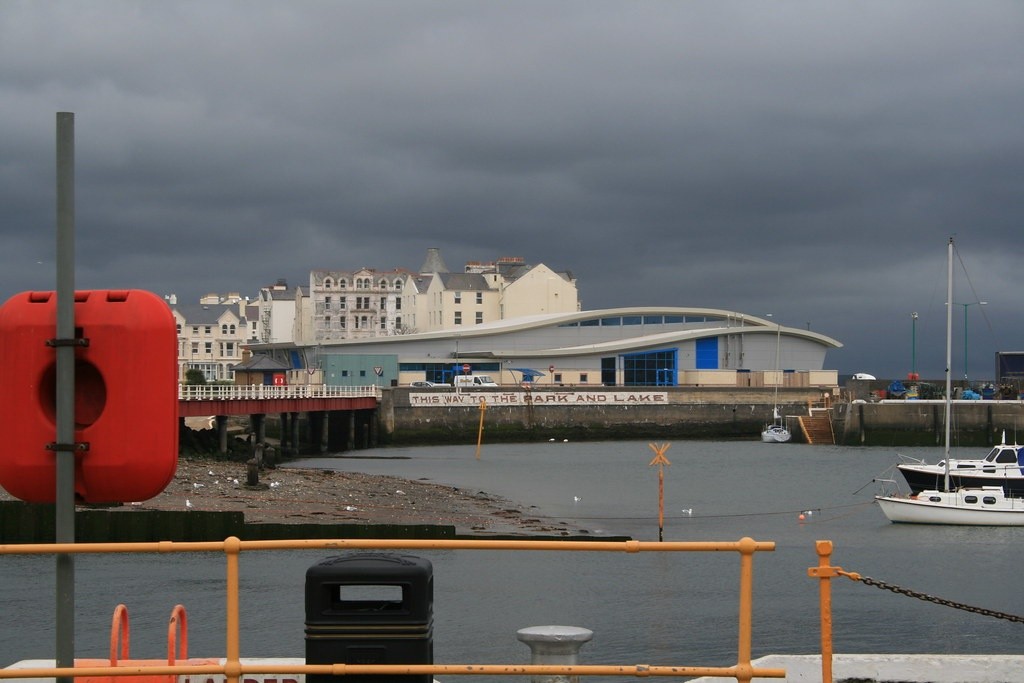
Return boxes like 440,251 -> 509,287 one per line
895,429 -> 1024,499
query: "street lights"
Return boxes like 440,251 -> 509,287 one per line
945,301 -> 991,381
910,312 -> 921,381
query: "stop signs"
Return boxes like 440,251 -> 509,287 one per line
462,364 -> 471,373
548,365 -> 554,373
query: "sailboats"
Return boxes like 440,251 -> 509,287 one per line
873,236 -> 1024,528
759,323 -> 794,443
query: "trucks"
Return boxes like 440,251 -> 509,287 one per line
454,374 -> 498,387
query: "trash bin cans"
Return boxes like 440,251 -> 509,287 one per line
305,553 -> 435,683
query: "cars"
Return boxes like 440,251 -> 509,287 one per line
409,380 -> 436,388
